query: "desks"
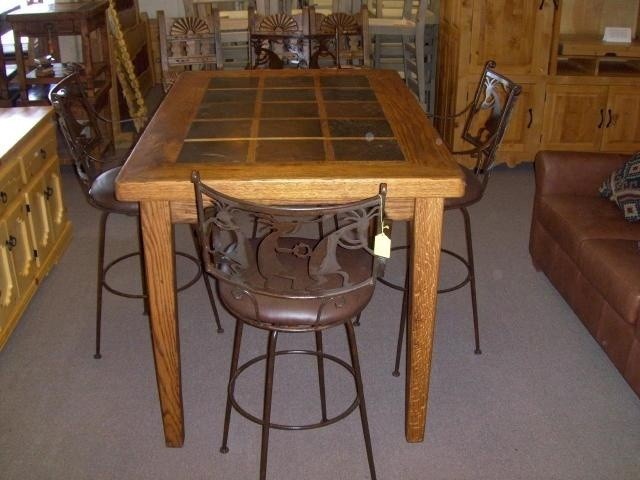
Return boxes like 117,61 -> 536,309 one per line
7,0 -> 143,178
114,68 -> 467,446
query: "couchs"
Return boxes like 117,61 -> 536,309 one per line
528,150 -> 640,398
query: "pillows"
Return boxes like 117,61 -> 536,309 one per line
598,149 -> 640,225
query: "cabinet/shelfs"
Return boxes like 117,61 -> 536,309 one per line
0,106 -> 74,351
436,0 -> 555,172
542,83 -> 640,154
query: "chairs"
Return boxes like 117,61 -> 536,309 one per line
48,71 -> 224,360
350,60 -> 523,377
368,0 -> 429,108
250,33 -> 341,240
190,170 -> 394,480
